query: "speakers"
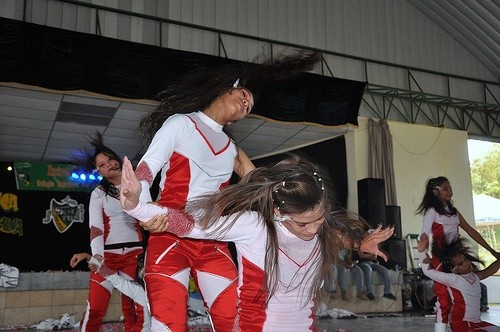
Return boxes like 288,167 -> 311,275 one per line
411,279 -> 436,312
377,239 -> 407,271
357,177 -> 387,229
386,205 -> 403,240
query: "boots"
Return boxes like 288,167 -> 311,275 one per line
434,323 -> 448,332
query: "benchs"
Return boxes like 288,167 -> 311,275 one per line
0,268 -> 404,327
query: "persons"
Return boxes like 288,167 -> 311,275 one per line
337,248 -> 368,301
414,177 -> 500,332
119,150 -> 394,332
351,228 -> 396,300
79,127 -> 143,332
325,274 -> 337,299
134,43 -> 324,332
70,253 -> 151,332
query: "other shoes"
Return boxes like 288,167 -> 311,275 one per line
368,293 -> 375,300
384,293 -> 397,301
357,293 -> 368,300
342,290 -> 348,301
330,290 -> 339,299
